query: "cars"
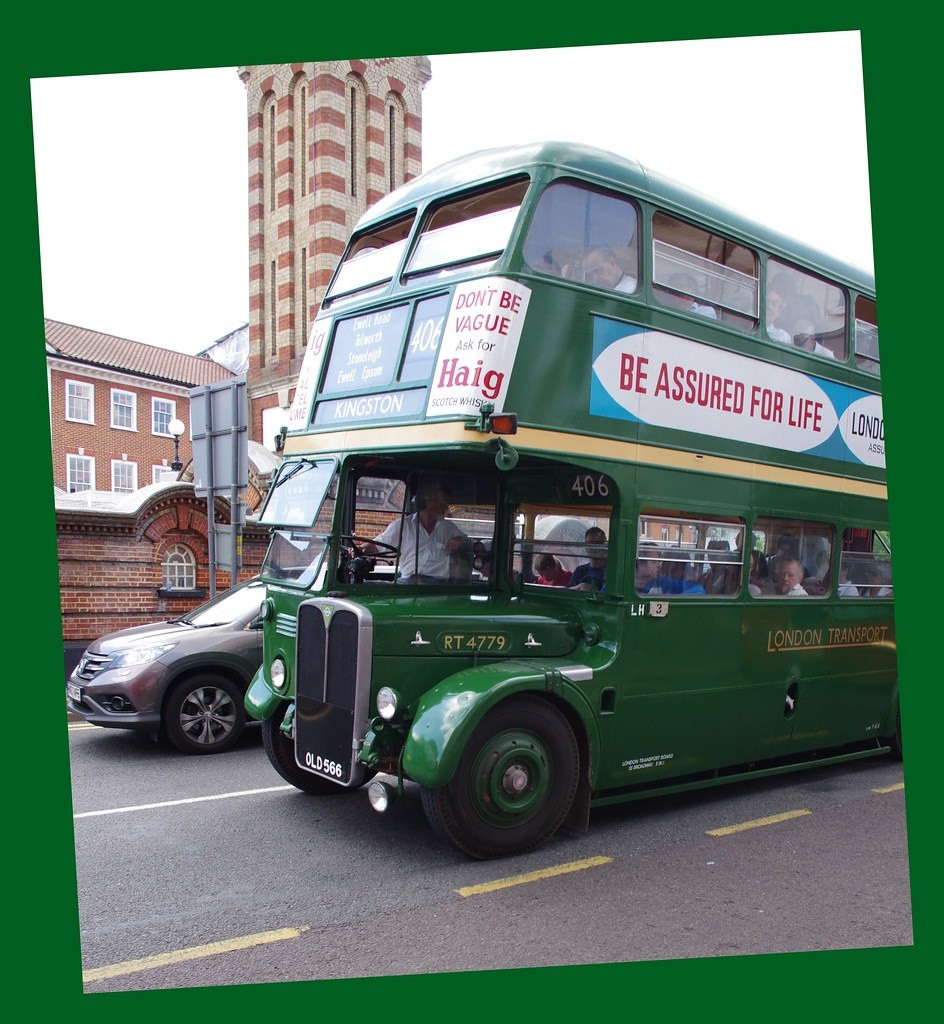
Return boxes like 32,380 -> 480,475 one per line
67,567 -> 312,755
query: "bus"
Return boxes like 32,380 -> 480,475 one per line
243,141 -> 902,861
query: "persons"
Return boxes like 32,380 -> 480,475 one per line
561,245 -> 880,374
534,527 -> 895,597
348,482 -> 473,584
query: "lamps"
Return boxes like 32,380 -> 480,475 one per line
167,418 -> 184,470
